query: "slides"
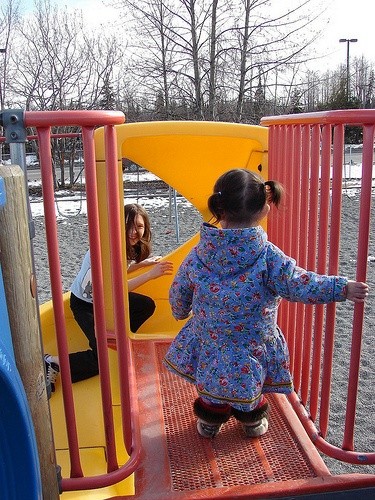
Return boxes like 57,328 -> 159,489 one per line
31,118 -> 272,500
0,175 -> 44,500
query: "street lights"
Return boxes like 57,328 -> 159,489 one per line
338,38 -> 358,131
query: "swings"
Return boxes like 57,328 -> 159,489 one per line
52,137 -> 83,219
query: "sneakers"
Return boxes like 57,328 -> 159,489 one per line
242,418 -> 268,436
196,418 -> 219,437
40,354 -> 59,399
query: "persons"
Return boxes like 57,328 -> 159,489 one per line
161,168 -> 370,438
44,203 -> 173,399
